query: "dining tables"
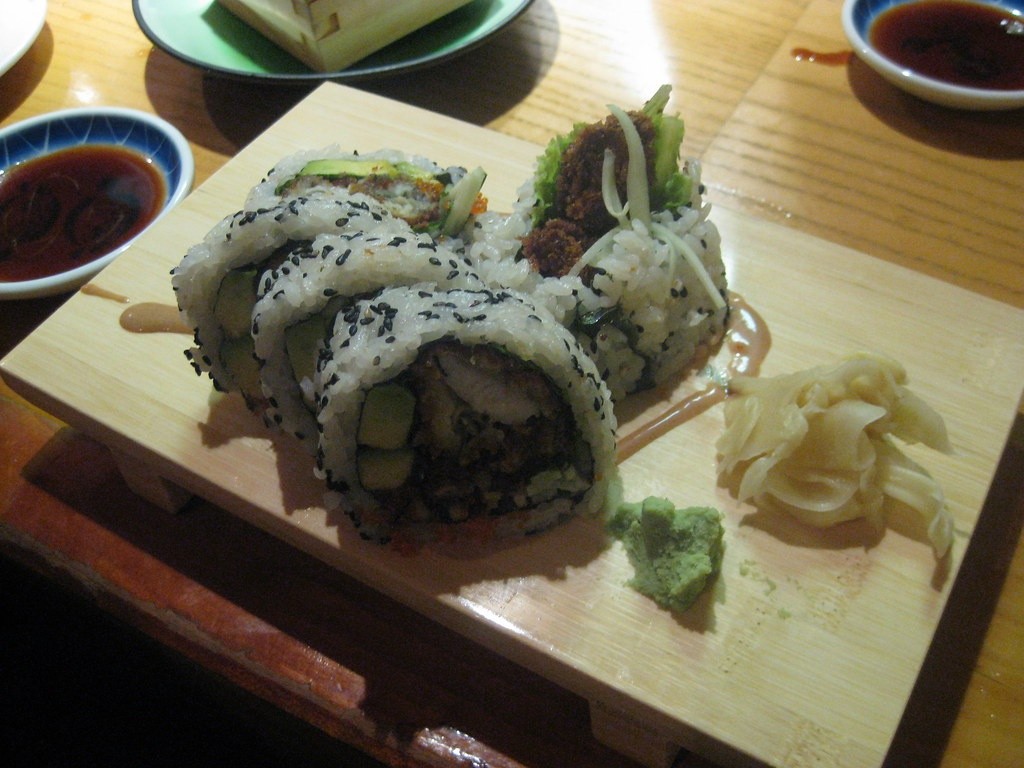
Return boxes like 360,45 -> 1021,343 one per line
0,2 -> 1024,766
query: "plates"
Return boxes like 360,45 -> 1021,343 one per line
0,0 -> 48,80
119,0 -> 539,78
0,78 -> 1024,768
0,103 -> 200,302
839,0 -> 1024,114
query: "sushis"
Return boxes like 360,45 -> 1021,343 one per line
172,83 -> 733,566
